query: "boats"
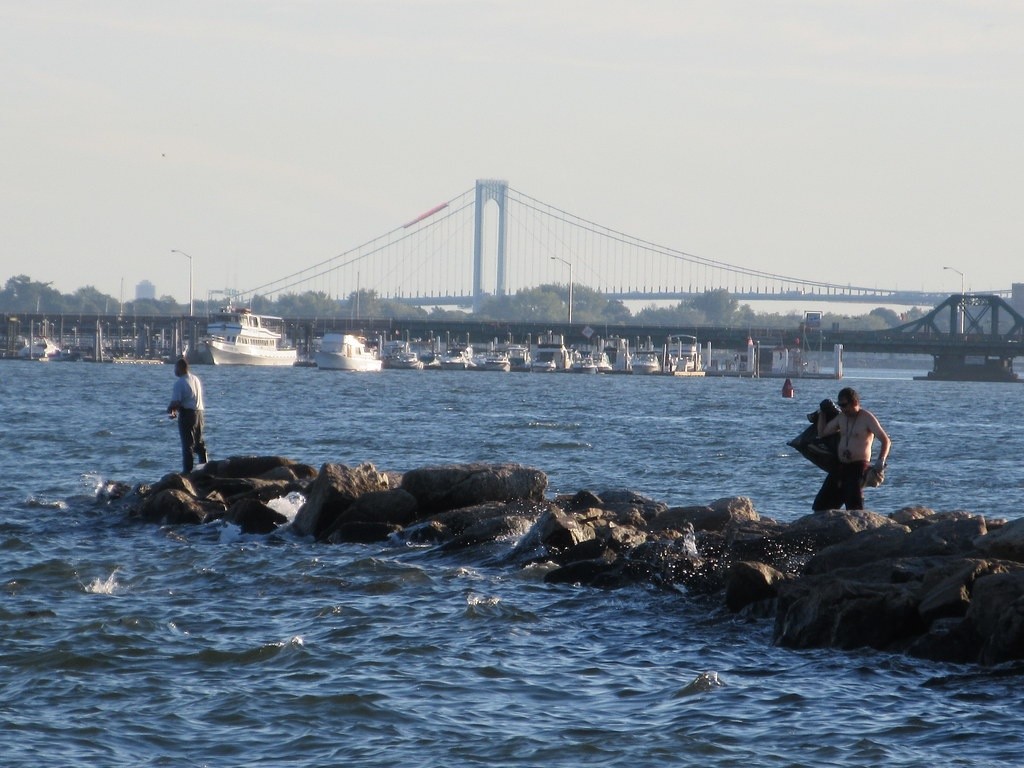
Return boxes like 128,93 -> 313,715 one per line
484,349 -> 511,371
629,353 -> 659,375
385,330 -> 661,369
667,334 -> 697,370
202,305 -> 297,366
571,356 -> 599,375
22,337 -> 61,358
313,333 -> 383,371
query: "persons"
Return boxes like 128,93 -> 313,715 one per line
813,387 -> 891,511
168,359 -> 206,477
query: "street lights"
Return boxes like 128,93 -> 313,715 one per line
944,266 -> 964,333
171,249 -> 193,316
551,256 -> 572,323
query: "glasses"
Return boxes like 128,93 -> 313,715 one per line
837,402 -> 852,408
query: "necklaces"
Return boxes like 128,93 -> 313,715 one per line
842,414 -> 857,460
